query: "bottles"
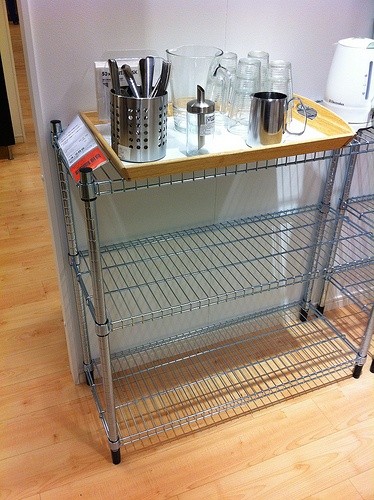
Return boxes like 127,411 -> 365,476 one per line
187,85 -> 217,155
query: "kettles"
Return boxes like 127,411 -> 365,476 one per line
323,37 -> 374,131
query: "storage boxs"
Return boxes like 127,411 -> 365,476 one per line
95,58 -> 142,122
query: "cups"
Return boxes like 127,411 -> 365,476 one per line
165,45 -> 231,133
212,51 -> 293,133
245,91 -> 307,148
